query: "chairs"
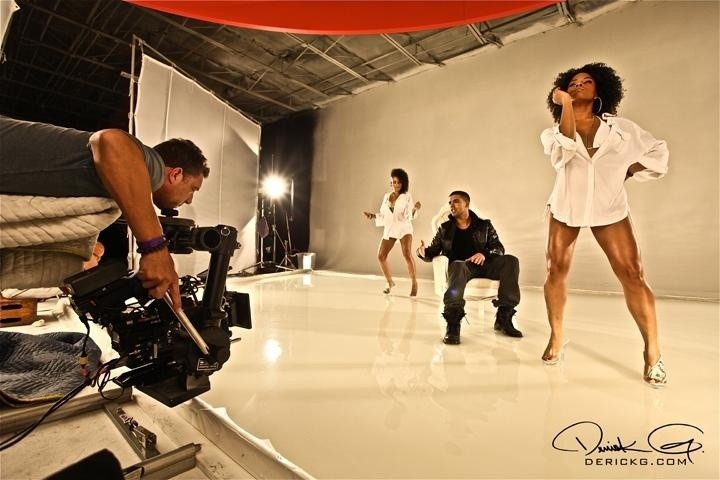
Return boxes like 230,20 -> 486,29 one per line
432,202 -> 519,329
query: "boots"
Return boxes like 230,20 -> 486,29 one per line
495,307 -> 522,337
442,310 -> 466,345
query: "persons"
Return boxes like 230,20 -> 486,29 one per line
1,112 -> 211,310
540,59 -> 671,391
363,167 -> 422,297
416,190 -> 524,345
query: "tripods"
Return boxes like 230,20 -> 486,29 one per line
275,240 -> 297,272
271,154 -> 296,267
238,194 -> 296,273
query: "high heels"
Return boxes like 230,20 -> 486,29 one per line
543,336 -> 570,365
643,350 -> 667,388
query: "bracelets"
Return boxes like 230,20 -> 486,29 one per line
136,240 -> 170,254
626,168 -> 633,177
136,233 -> 167,249
